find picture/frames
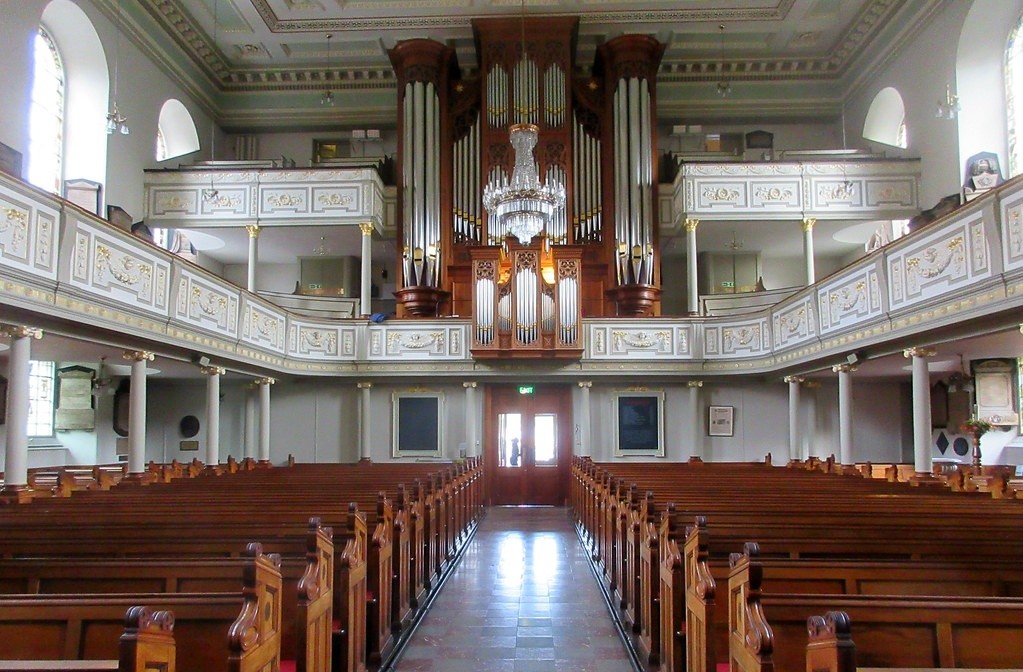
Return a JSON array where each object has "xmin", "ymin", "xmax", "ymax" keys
[
  {"xmin": 708, "ymin": 405, "xmax": 734, "ymax": 438},
  {"xmin": 610, "ymin": 386, "xmax": 666, "ymax": 458},
  {"xmin": 391, "ymin": 390, "xmax": 445, "ymax": 458}
]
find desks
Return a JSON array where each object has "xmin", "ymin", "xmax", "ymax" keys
[
  {"xmin": 349, "ymin": 138, "xmax": 384, "ymax": 158},
  {"xmin": 669, "ymin": 134, "xmax": 705, "ymax": 151}
]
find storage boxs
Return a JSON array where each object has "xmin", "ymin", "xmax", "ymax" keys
[
  {"xmin": 366, "ymin": 129, "xmax": 380, "ymax": 138},
  {"xmin": 689, "ymin": 125, "xmax": 703, "ymax": 134},
  {"xmin": 672, "ymin": 124, "xmax": 687, "ymax": 134},
  {"xmin": 705, "ymin": 134, "xmax": 720, "ymax": 151},
  {"xmin": 352, "ymin": 129, "xmax": 366, "ymax": 138}
]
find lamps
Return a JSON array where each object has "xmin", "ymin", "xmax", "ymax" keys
[
  {"xmin": 381, "ymin": 268, "xmax": 388, "ymax": 279},
  {"xmin": 723, "ymin": 231, "xmax": 743, "ymax": 251},
  {"xmin": 312, "ymin": 236, "xmax": 330, "ymax": 256},
  {"xmin": 717, "ymin": 23, "xmax": 732, "ymax": 98},
  {"xmin": 320, "ymin": 34, "xmax": 335, "ymax": 107},
  {"xmin": 104, "ymin": 0, "xmax": 129, "ymax": 137},
  {"xmin": 935, "ymin": 83, "xmax": 963, "ymax": 120},
  {"xmin": 846, "ymin": 350, "xmax": 867, "ymax": 366},
  {"xmin": 200, "ymin": 0, "xmax": 220, "ymax": 205},
  {"xmin": 480, "ymin": 0, "xmax": 567, "ymax": 249},
  {"xmin": 89, "ymin": 355, "xmax": 116, "ymax": 398}
]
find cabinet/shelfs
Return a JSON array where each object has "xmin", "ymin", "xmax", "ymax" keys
[
  {"xmin": 697, "ymin": 250, "xmax": 762, "ymax": 302},
  {"xmin": 296, "ymin": 255, "xmax": 361, "ymax": 298}
]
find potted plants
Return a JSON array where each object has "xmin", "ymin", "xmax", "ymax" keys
[{"xmin": 959, "ymin": 418, "xmax": 991, "ymax": 465}]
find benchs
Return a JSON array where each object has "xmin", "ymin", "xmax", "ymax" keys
[
  {"xmin": 569, "ymin": 454, "xmax": 1023, "ymax": 672},
  {"xmin": 0, "ymin": 449, "xmax": 484, "ymax": 672}
]
